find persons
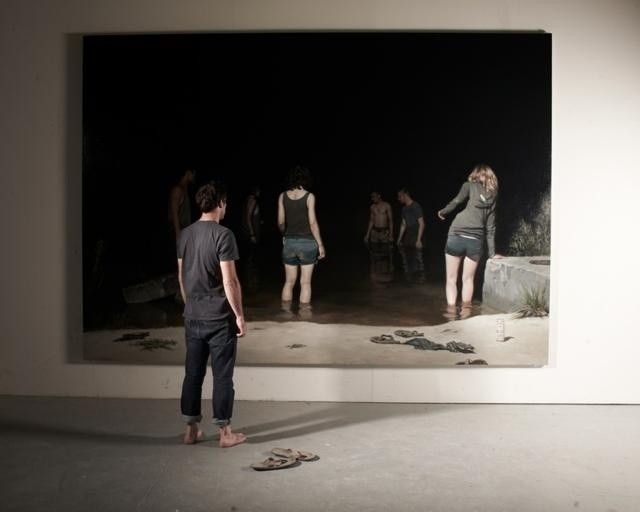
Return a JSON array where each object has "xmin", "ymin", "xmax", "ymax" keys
[
  {"xmin": 364, "ymin": 190, "xmax": 395, "ymax": 243},
  {"xmin": 241, "ymin": 184, "xmax": 264, "ymax": 246},
  {"xmin": 396, "ymin": 188, "xmax": 425, "ymax": 248},
  {"xmin": 167, "ymin": 165, "xmax": 195, "ymax": 238},
  {"xmin": 174, "ymin": 177, "xmax": 247, "ymax": 448},
  {"xmin": 438, "ymin": 162, "xmax": 504, "ymax": 323},
  {"xmin": 277, "ymin": 164, "xmax": 326, "ymax": 303}
]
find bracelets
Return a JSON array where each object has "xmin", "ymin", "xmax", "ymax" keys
[{"xmin": 250, "ymin": 233, "xmax": 256, "ymax": 237}]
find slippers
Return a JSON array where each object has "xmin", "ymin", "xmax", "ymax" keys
[
  {"xmin": 251, "ymin": 455, "xmax": 297, "ymax": 471},
  {"xmin": 395, "ymin": 330, "xmax": 424, "ymax": 337},
  {"xmin": 371, "ymin": 334, "xmax": 400, "ymax": 344},
  {"xmin": 271, "ymin": 447, "xmax": 316, "ymax": 461}
]
